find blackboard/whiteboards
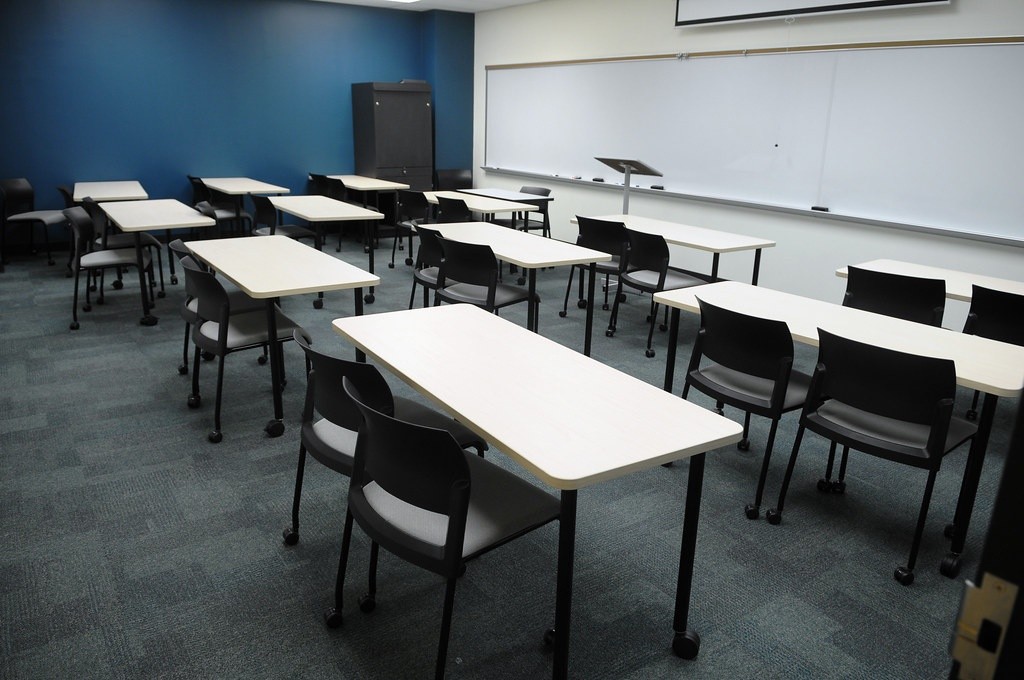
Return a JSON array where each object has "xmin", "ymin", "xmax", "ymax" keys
[{"xmin": 480, "ymin": 37, "xmax": 1024, "ymax": 249}]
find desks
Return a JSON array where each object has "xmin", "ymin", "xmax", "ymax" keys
[
  {"xmin": 182, "ymin": 234, "xmax": 381, "ymax": 439},
  {"xmin": 330, "ymin": 303, "xmax": 745, "ymax": 679},
  {"xmin": 201, "ymin": 177, "xmax": 290, "ymax": 238},
  {"xmin": 73, "ymin": 180, "xmax": 149, "ymax": 201},
  {"xmin": 653, "ymin": 281, "xmax": 1024, "ymax": 580},
  {"xmin": 309, "ymin": 175, "xmax": 410, "ymax": 253},
  {"xmin": 410, "ymin": 221, "xmax": 613, "ymax": 358},
  {"xmin": 267, "ymin": 196, "xmax": 384, "ymax": 309},
  {"xmin": 98, "ymin": 197, "xmax": 216, "ymax": 327},
  {"xmin": 423, "ymin": 187, "xmax": 554, "ymax": 286},
  {"xmin": 571, "ymin": 214, "xmax": 776, "ymax": 328},
  {"xmin": 836, "ymin": 258, "xmax": 1024, "ymax": 304}
]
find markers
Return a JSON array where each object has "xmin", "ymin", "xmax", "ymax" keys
[
  {"xmin": 636, "ymin": 185, "xmax": 650, "ymax": 188},
  {"xmin": 491, "ymin": 167, "xmax": 499, "ymax": 170},
  {"xmin": 571, "ymin": 176, "xmax": 582, "ymax": 179}
]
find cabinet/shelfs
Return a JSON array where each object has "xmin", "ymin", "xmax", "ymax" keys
[{"xmin": 352, "ymin": 82, "xmax": 434, "ymax": 238}]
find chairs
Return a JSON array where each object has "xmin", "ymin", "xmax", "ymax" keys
[
  {"xmin": 1, "ymin": 167, "xmax": 707, "ymax": 442},
  {"xmin": 324, "ymin": 375, "xmax": 563, "ymax": 679},
  {"xmin": 962, "ymin": 283, "xmax": 1024, "ymax": 421},
  {"xmin": 842, "ymin": 266, "xmax": 947, "ymax": 329},
  {"xmin": 659, "ymin": 294, "xmax": 836, "ymax": 520},
  {"xmin": 766, "ymin": 325, "xmax": 976, "ymax": 589},
  {"xmin": 281, "ymin": 326, "xmax": 490, "ymax": 613}
]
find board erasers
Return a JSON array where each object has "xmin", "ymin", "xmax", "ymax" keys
[
  {"xmin": 812, "ymin": 205, "xmax": 829, "ymax": 212},
  {"xmin": 650, "ymin": 184, "xmax": 664, "ymax": 190},
  {"xmin": 593, "ymin": 177, "xmax": 605, "ymax": 182}
]
